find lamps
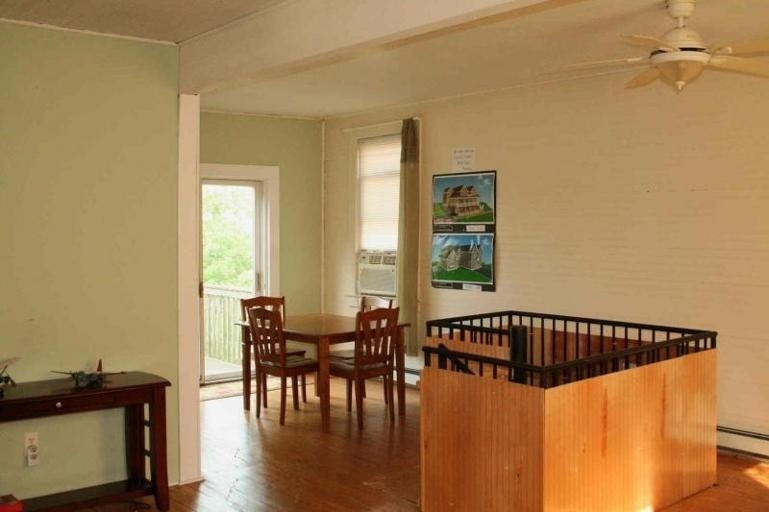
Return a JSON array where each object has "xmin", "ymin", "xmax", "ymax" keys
[{"xmin": 650, "ymin": 50, "xmax": 711, "ymax": 92}]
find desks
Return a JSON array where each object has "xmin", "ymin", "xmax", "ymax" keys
[
  {"xmin": 2, "ymin": 369, "xmax": 170, "ymax": 511},
  {"xmin": 234, "ymin": 314, "xmax": 412, "ymax": 426}
]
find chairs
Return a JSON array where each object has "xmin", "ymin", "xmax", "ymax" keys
[{"xmin": 236, "ymin": 292, "xmax": 401, "ymax": 431}]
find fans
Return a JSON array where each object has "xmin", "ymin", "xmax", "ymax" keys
[{"xmin": 551, "ymin": 0, "xmax": 769, "ymax": 102}]
